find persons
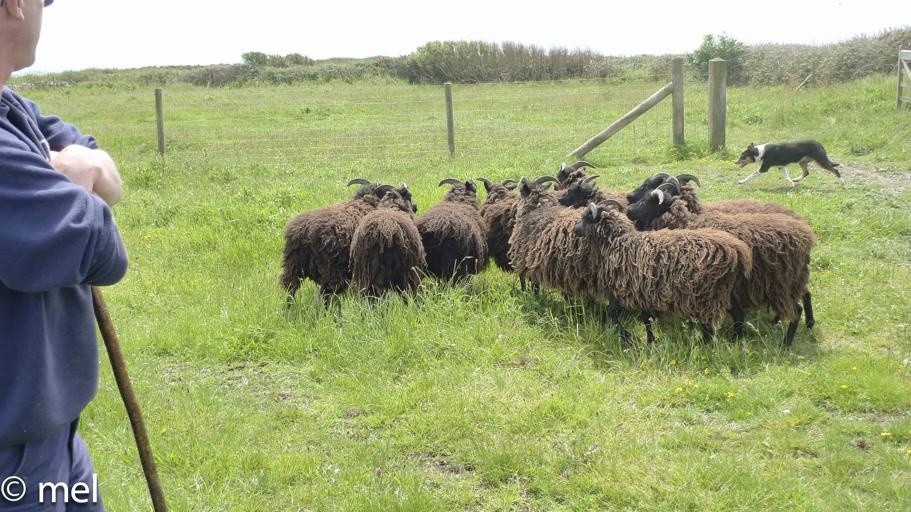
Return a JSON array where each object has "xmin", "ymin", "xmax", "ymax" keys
[{"xmin": 1, "ymin": 0, "xmax": 128, "ymax": 511}]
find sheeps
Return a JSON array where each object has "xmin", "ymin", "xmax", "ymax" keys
[
  {"xmin": 411, "ymin": 177, "xmax": 490, "ymax": 284},
  {"xmin": 349, "ymin": 182, "xmax": 429, "ymax": 309},
  {"xmin": 277, "ymin": 178, "xmax": 385, "ymax": 322},
  {"xmin": 477, "ymin": 161, "xmax": 818, "ymax": 347}
]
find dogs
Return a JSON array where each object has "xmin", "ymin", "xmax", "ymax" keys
[{"xmin": 732, "ymin": 136, "xmax": 844, "ymax": 190}]
